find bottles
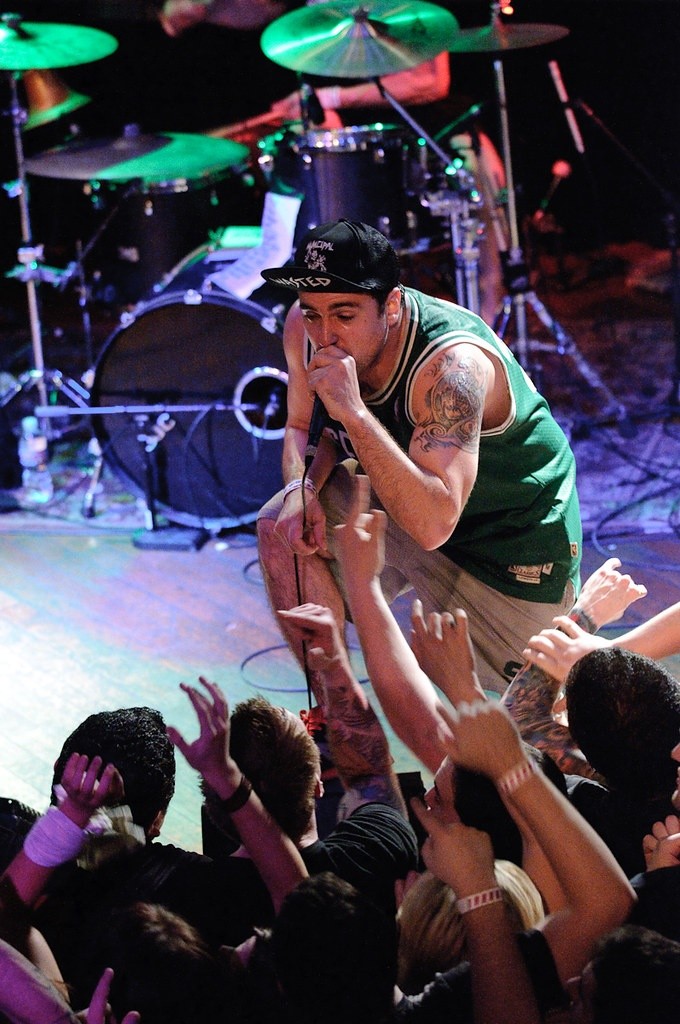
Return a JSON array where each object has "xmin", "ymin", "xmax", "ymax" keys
[{"xmin": 17, "ymin": 415, "xmax": 54, "ymax": 504}]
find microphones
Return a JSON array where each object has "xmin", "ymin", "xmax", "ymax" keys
[
  {"xmin": 548, "ymin": 60, "xmax": 585, "ymax": 155},
  {"xmin": 297, "ymin": 72, "xmax": 325, "ymax": 125},
  {"xmin": 305, "ymin": 393, "xmax": 328, "ymax": 468},
  {"xmin": 532, "ymin": 160, "xmax": 570, "ymax": 221}
]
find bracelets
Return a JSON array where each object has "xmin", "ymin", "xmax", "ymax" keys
[
  {"xmin": 282, "ymin": 479, "xmax": 319, "ymax": 503},
  {"xmin": 220, "ymin": 774, "xmax": 252, "ymax": 811},
  {"xmin": 457, "ymin": 886, "xmax": 501, "ymax": 916},
  {"xmin": 497, "ymin": 755, "xmax": 537, "ymax": 797},
  {"xmin": 332, "ymin": 86, "xmax": 341, "ymax": 108}
]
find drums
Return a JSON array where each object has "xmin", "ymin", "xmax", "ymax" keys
[
  {"xmin": 90, "ymin": 159, "xmax": 254, "ymax": 305},
  {"xmin": 263, "ymin": 125, "xmax": 465, "ymax": 258},
  {"xmin": 88, "ymin": 251, "xmax": 329, "ymax": 530}
]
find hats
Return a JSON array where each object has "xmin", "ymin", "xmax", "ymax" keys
[{"xmin": 258, "ymin": 216, "xmax": 400, "ymax": 297}]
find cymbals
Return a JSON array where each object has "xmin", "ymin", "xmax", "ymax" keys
[
  {"xmin": 0, "ymin": 21, "xmax": 119, "ymax": 71},
  {"xmin": 444, "ymin": 23, "xmax": 569, "ymax": 52},
  {"xmin": 22, "ymin": 127, "xmax": 251, "ymax": 178},
  {"xmin": 260, "ymin": 0, "xmax": 460, "ymax": 79}
]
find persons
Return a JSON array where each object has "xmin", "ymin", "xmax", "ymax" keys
[
  {"xmin": 160, "ymin": 0, "xmax": 511, "ymax": 330},
  {"xmin": 0, "ymin": 556, "xmax": 680, "ymax": 1024},
  {"xmin": 258, "ymin": 222, "xmax": 584, "ymax": 783}
]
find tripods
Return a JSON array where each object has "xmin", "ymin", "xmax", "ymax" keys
[
  {"xmin": 492, "ymin": 54, "xmax": 640, "ymax": 440},
  {"xmin": 0, "ymin": 73, "xmax": 95, "ymax": 456}
]
